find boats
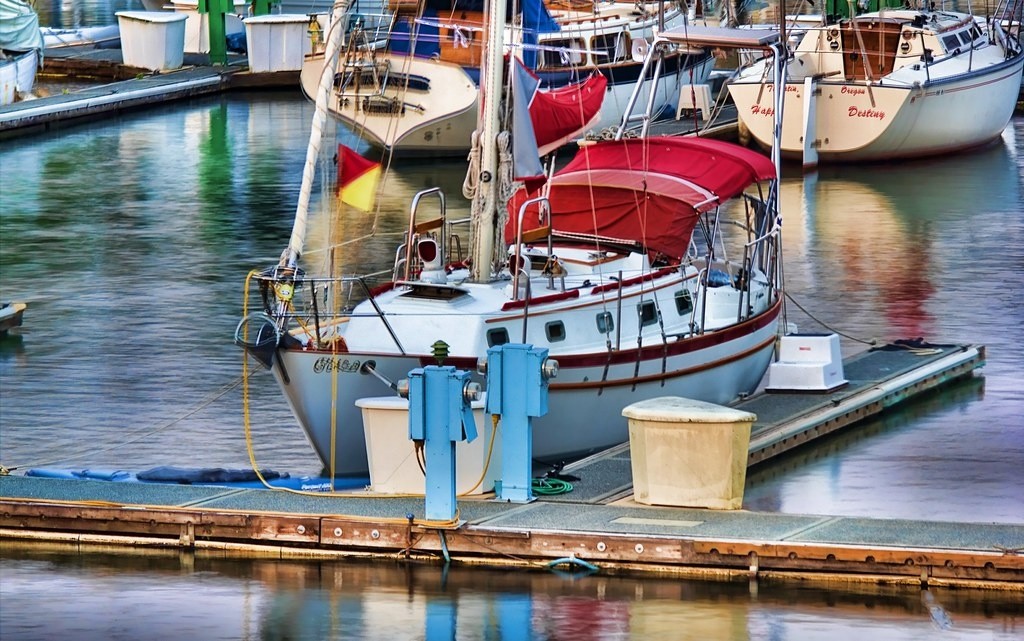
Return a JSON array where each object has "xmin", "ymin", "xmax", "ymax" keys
[
  {"xmin": 291, "ymin": 0, "xmax": 1024, "ymax": 165},
  {"xmin": 0, "ymin": 0, "xmax": 46, "ymax": 108},
  {"xmin": 234, "ymin": 0, "xmax": 789, "ymax": 485},
  {"xmin": 37, "ymin": 23, "xmax": 122, "ymax": 59}
]
